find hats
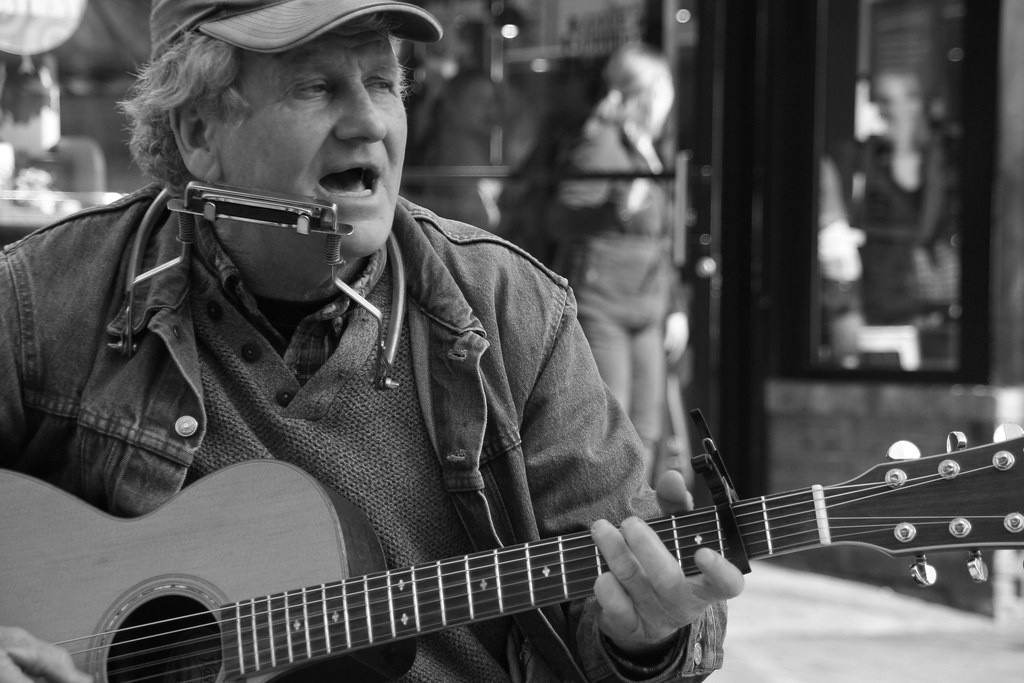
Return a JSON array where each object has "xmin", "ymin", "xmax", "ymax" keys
[{"xmin": 150, "ymin": 0, "xmax": 443, "ymax": 64}]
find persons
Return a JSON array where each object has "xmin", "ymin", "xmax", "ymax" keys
[
  {"xmin": 820, "ymin": 64, "xmax": 949, "ymax": 370},
  {"xmin": 0, "ymin": 0, "xmax": 744, "ymax": 683}
]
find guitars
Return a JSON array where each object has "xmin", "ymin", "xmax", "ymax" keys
[{"xmin": 0, "ymin": 421, "xmax": 1024, "ymax": 683}]
